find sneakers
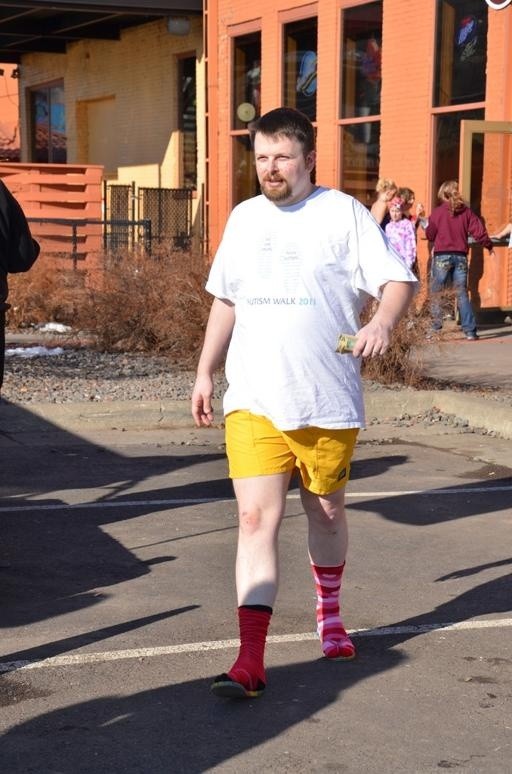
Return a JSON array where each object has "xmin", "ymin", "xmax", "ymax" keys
[{"xmin": 465, "ymin": 329, "xmax": 482, "ymax": 342}]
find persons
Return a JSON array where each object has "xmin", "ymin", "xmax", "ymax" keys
[
  {"xmin": 369, "ymin": 176, "xmax": 399, "ymax": 231},
  {"xmin": 488, "ymin": 221, "xmax": 511, "ymax": 240},
  {"xmin": 0, "ymin": 175, "xmax": 43, "ymax": 388},
  {"xmin": 424, "ymin": 179, "xmax": 498, "ymax": 341},
  {"xmin": 395, "ymin": 186, "xmax": 427, "ymax": 230},
  {"xmin": 190, "ymin": 104, "xmax": 420, "ymax": 701},
  {"xmin": 381, "ymin": 196, "xmax": 419, "ymax": 269}
]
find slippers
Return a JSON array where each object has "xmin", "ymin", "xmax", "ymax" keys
[
  {"xmin": 210, "ymin": 669, "xmax": 268, "ymax": 700},
  {"xmin": 318, "ymin": 635, "xmax": 358, "ymax": 664}
]
